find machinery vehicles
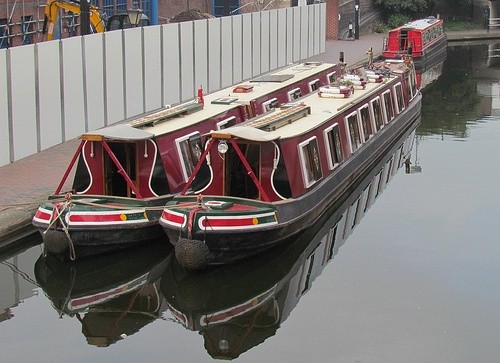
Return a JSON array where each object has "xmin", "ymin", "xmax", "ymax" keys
[{"xmin": 41, "ymin": 0, "xmax": 150, "ymax": 43}]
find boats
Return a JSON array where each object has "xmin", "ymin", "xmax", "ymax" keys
[
  {"xmin": 33, "ymin": 237, "xmax": 176, "ymax": 349},
  {"xmin": 31, "ymin": 60, "xmax": 348, "ymax": 262},
  {"xmin": 411, "ymin": 34, "xmax": 449, "ymax": 90},
  {"xmin": 159, "ymin": 115, "xmax": 424, "ymax": 361},
  {"xmin": 381, "ymin": 14, "xmax": 447, "ymax": 61},
  {"xmin": 159, "ymin": 58, "xmax": 423, "ymax": 275}
]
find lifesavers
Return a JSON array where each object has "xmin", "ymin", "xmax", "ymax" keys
[
  {"xmin": 353, "ymin": 86, "xmax": 366, "ymax": 90},
  {"xmin": 318, "ymin": 86, "xmax": 354, "ymax": 93},
  {"xmin": 367, "ymin": 79, "xmax": 383, "ymax": 83},
  {"xmin": 318, "ymin": 92, "xmax": 351, "ymax": 98},
  {"xmin": 338, "ymin": 75, "xmax": 367, "ymax": 85},
  {"xmin": 365, "ymin": 70, "xmax": 383, "ymax": 79}
]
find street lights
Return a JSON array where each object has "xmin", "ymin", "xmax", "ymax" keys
[{"xmin": 127, "ymin": 1, "xmax": 144, "ymax": 28}]
